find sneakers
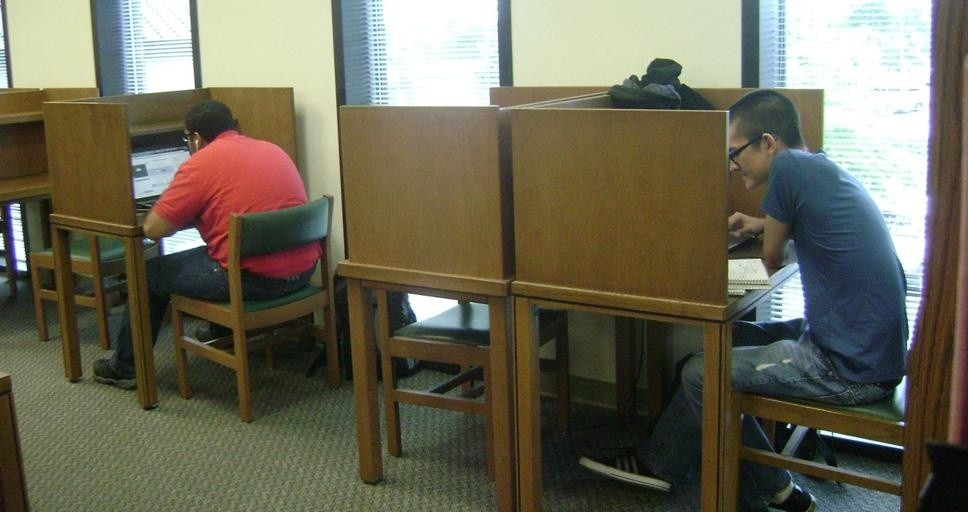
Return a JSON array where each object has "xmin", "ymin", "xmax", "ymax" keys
[
  {"xmin": 579, "ymin": 453, "xmax": 672, "ymax": 494},
  {"xmin": 92, "ymin": 358, "xmax": 136, "ymax": 389},
  {"xmin": 195, "ymin": 326, "xmax": 213, "ymax": 342},
  {"xmin": 760, "ymin": 481, "xmax": 816, "ymax": 512}
]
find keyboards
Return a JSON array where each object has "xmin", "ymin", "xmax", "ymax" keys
[{"xmin": 728, "ymin": 236, "xmax": 750, "ymax": 254}]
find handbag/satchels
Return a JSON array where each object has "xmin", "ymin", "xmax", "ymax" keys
[{"xmin": 333, "ymin": 271, "xmax": 421, "ymax": 380}]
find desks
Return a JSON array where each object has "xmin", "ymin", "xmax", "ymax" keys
[
  {"xmin": 0, "ymin": 84, "xmax": 298, "ymax": 391},
  {"xmin": 331, "ymin": 84, "xmax": 826, "ymax": 510}
]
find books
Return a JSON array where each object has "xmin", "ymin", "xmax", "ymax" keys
[{"xmin": 727, "ymin": 257, "xmax": 772, "ymax": 297}]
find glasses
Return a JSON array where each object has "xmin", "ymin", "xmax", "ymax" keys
[
  {"xmin": 727, "ymin": 134, "xmax": 763, "ymax": 162},
  {"xmin": 182, "ymin": 133, "xmax": 193, "ymax": 142}
]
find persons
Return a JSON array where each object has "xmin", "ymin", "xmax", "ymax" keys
[
  {"xmin": 578, "ymin": 87, "xmax": 909, "ymax": 512},
  {"xmin": 92, "ymin": 99, "xmax": 323, "ymax": 390}
]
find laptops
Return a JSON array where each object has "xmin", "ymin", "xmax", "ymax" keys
[{"xmin": 131, "ymin": 144, "xmax": 191, "ymax": 209}]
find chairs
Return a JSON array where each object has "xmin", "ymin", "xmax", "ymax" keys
[
  {"xmin": 167, "ymin": 191, "xmax": 344, "ymax": 424},
  {"xmin": 732, "ymin": 224, "xmax": 932, "ymax": 512}
]
